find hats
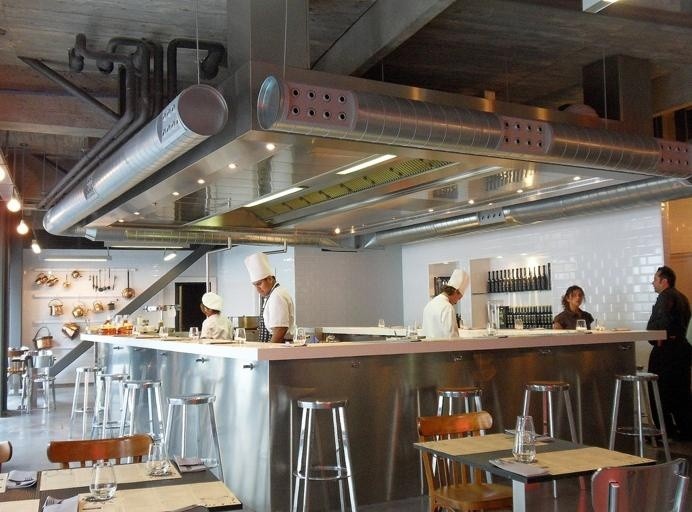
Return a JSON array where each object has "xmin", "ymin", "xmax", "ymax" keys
[
  {"xmin": 447, "ymin": 269, "xmax": 469, "ymax": 295},
  {"xmin": 202, "ymin": 292, "xmax": 222, "ymax": 310},
  {"xmin": 244, "ymin": 252, "xmax": 274, "ymax": 282}
]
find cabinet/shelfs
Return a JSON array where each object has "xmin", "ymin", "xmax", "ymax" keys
[{"xmin": 471, "ymin": 251, "xmax": 553, "ymax": 329}]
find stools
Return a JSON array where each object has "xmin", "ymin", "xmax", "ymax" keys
[
  {"xmin": 165, "ymin": 394, "xmax": 224, "ymax": 481},
  {"xmin": 91, "ymin": 374, "xmax": 130, "ymax": 439},
  {"xmin": 523, "ymin": 381, "xmax": 586, "ymax": 498},
  {"xmin": 70, "ymin": 365, "xmax": 101, "ymax": 433},
  {"xmin": 292, "ymin": 398, "xmax": 359, "ymax": 512},
  {"xmin": 119, "ymin": 380, "xmax": 165, "ymax": 464},
  {"xmin": 431, "ymin": 386, "xmax": 494, "ymax": 483},
  {"xmin": 609, "ymin": 373, "xmax": 671, "ymax": 462}
]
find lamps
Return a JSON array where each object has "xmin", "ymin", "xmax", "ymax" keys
[
  {"xmin": 0, "ymin": 131, "xmax": 41, "ymax": 253},
  {"xmin": 42, "ymin": 246, "xmax": 111, "ymax": 261},
  {"xmin": 162, "ymin": 248, "xmax": 176, "ymax": 261}
]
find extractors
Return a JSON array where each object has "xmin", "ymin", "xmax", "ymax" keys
[{"xmin": 250, "ymin": 155, "xmax": 455, "ymax": 223}]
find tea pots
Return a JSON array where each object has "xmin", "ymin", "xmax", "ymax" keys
[{"xmin": 72, "ymin": 300, "xmax": 104, "ymax": 318}]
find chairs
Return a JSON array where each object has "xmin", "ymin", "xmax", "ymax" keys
[
  {"xmin": 47, "ymin": 434, "xmax": 154, "ymax": 469},
  {"xmin": 591, "ymin": 458, "xmax": 690, "ymax": 512},
  {"xmin": 416, "ymin": 411, "xmax": 513, "ymax": 512},
  {"xmin": 21, "ymin": 349, "xmax": 56, "ymax": 411},
  {"xmin": 0, "ymin": 441, "xmax": 12, "ymax": 472},
  {"xmin": 7, "ymin": 350, "xmax": 27, "ymax": 380}
]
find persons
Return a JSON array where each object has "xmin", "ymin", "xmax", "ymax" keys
[
  {"xmin": 198, "ymin": 291, "xmax": 236, "ymax": 341},
  {"xmin": 421, "ymin": 269, "xmax": 469, "ymax": 339},
  {"xmin": 551, "ymin": 284, "xmax": 596, "ymax": 329},
  {"xmin": 645, "ymin": 266, "xmax": 691, "ymax": 447},
  {"xmin": 244, "ymin": 252, "xmax": 297, "ymax": 343}
]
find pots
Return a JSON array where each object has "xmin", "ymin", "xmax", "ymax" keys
[
  {"xmin": 34, "ymin": 336, "xmax": 53, "ymax": 348},
  {"xmin": 35, "ymin": 270, "xmax": 83, "ymax": 288},
  {"xmin": 49, "ymin": 305, "xmax": 64, "ymax": 316},
  {"xmin": 122, "ymin": 271, "xmax": 135, "ymax": 300}
]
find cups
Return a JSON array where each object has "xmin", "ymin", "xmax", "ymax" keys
[
  {"xmin": 314, "ymin": 327, "xmax": 323, "ymax": 341},
  {"xmin": 378, "ymin": 319, "xmax": 385, "ymax": 328},
  {"xmin": 595, "ymin": 317, "xmax": 605, "ymax": 330},
  {"xmin": 189, "ymin": 327, "xmax": 199, "ymax": 339},
  {"xmin": 575, "ymin": 319, "xmax": 587, "ymax": 331},
  {"xmin": 159, "ymin": 327, "xmax": 169, "ymax": 339},
  {"xmin": 234, "ymin": 328, "xmax": 247, "ymax": 341},
  {"xmin": 486, "ymin": 322, "xmax": 497, "ymax": 336},
  {"xmin": 406, "ymin": 321, "xmax": 418, "ymax": 339},
  {"xmin": 293, "ymin": 327, "xmax": 306, "ymax": 347}
]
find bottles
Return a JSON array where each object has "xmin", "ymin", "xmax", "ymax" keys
[
  {"xmin": 116, "ymin": 314, "xmax": 129, "ymax": 325},
  {"xmin": 145, "ymin": 442, "xmax": 170, "ymax": 475},
  {"xmin": 499, "ymin": 307, "xmax": 552, "ymax": 328},
  {"xmin": 456, "ymin": 313, "xmax": 461, "ymax": 328},
  {"xmin": 89, "ymin": 462, "xmax": 119, "ymax": 500},
  {"xmin": 486, "ymin": 262, "xmax": 550, "ymax": 293},
  {"xmin": 512, "ymin": 416, "xmax": 536, "ymax": 463},
  {"xmin": 435, "ymin": 277, "xmax": 450, "ymax": 296}
]
